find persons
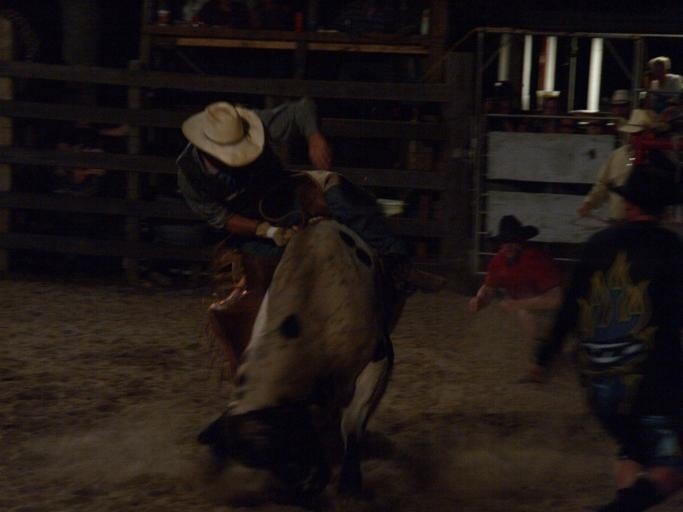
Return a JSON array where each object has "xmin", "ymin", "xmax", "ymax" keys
[
  {"xmin": 50, "ymin": 0, "xmax": 682, "ymax": 384},
  {"xmin": 172, "ymin": 89, "xmax": 447, "ymax": 313},
  {"xmin": 513, "ymin": 174, "xmax": 683, "ymax": 511}
]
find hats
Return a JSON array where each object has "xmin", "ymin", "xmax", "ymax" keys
[
  {"xmin": 181, "ymin": 103, "xmax": 266, "ymax": 166},
  {"xmin": 609, "ymin": 89, "xmax": 630, "ymax": 104},
  {"xmin": 606, "ymin": 163, "xmax": 681, "ymax": 206},
  {"xmin": 617, "ymin": 108, "xmax": 670, "ymax": 134},
  {"xmin": 486, "ymin": 215, "xmax": 539, "ymax": 243}
]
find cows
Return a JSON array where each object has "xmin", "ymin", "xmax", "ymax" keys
[{"xmin": 198, "ymin": 214, "xmax": 394, "ymax": 512}]
